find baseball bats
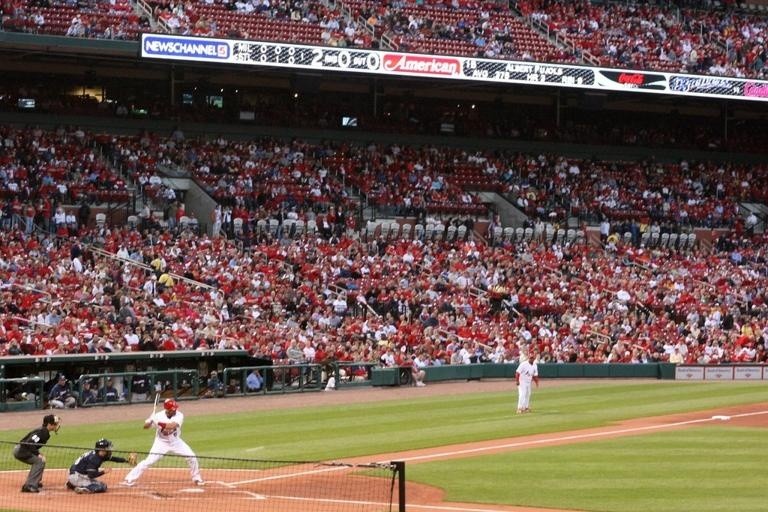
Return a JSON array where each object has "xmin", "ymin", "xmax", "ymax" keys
[{"xmin": 152, "ymin": 392, "xmax": 160, "ymax": 417}]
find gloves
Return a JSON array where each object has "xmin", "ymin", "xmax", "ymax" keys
[{"xmin": 145, "ymin": 413, "xmax": 159, "ymax": 427}]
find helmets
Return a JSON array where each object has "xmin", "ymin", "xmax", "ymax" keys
[
  {"xmin": 43, "ymin": 414, "xmax": 59, "ymax": 428},
  {"xmin": 94, "ymin": 439, "xmax": 114, "ymax": 452}
]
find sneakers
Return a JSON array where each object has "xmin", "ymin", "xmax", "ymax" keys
[
  {"xmin": 74, "ymin": 486, "xmax": 90, "ymax": 494},
  {"xmin": 124, "ymin": 480, "xmax": 137, "ymax": 487},
  {"xmin": 191, "ymin": 480, "xmax": 208, "ymax": 487},
  {"xmin": 22, "ymin": 482, "xmax": 42, "ymax": 493},
  {"xmin": 415, "ymin": 381, "xmax": 426, "ymax": 387},
  {"xmin": 516, "ymin": 408, "xmax": 531, "ymax": 414}
]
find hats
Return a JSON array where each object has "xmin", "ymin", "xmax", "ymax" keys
[
  {"xmin": 105, "ymin": 377, "xmax": 111, "ymax": 382},
  {"xmin": 163, "ymin": 399, "xmax": 179, "ymax": 410},
  {"xmin": 59, "ymin": 375, "xmax": 65, "ymax": 379},
  {"xmin": 529, "ymin": 352, "xmax": 536, "ymax": 359},
  {"xmin": 83, "ymin": 379, "xmax": 90, "ymax": 384}
]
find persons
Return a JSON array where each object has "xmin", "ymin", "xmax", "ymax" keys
[
  {"xmin": 515, "ymin": 354, "xmax": 539, "ymax": 414},
  {"xmin": 119, "ymin": 399, "xmax": 205, "ymax": 487},
  {"xmin": 0, "ymin": 1, "xmax": 768, "ymax": 410},
  {"xmin": 13, "ymin": 415, "xmax": 59, "ymax": 492},
  {"xmin": 67, "ymin": 438, "xmax": 129, "ymax": 494}
]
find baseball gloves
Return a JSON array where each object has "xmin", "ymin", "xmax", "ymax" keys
[{"xmin": 127, "ymin": 452, "xmax": 138, "ymax": 467}]
